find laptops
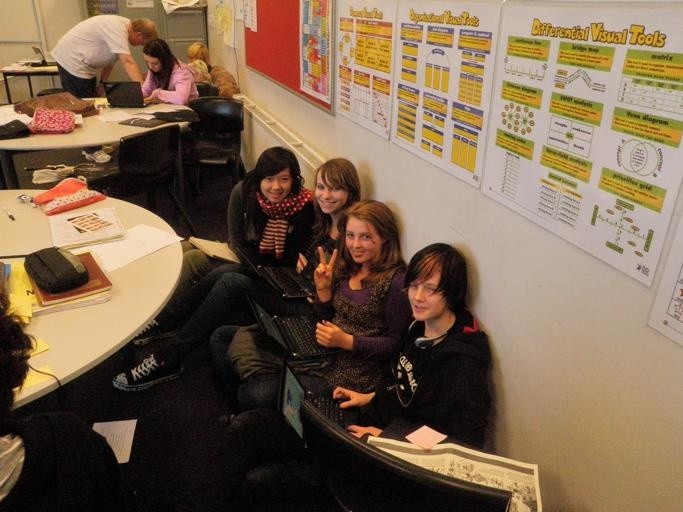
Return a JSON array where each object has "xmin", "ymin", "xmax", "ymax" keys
[
  {"xmin": 243, "ymin": 292, "xmax": 344, "ymax": 361},
  {"xmin": 234, "ymin": 246, "xmax": 317, "ymax": 298},
  {"xmin": 31, "ymin": 46, "xmax": 57, "ymax": 66},
  {"xmin": 102, "ymin": 81, "xmax": 150, "ymax": 108},
  {"xmin": 276, "ymin": 360, "xmax": 346, "ymax": 450}
]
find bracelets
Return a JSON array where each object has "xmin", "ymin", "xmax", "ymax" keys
[{"xmin": 100, "ymin": 79, "xmax": 107, "ymax": 85}]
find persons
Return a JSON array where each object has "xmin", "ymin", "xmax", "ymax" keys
[
  {"xmin": 119, "ymin": 38, "xmax": 199, "ymax": 178},
  {"xmin": 187, "ymin": 42, "xmax": 212, "ymax": 74},
  {"xmin": 197, "ymin": 242, "xmax": 491, "ymax": 510},
  {"xmin": 112, "ymin": 159, "xmax": 361, "ymax": 393},
  {"xmin": 50, "ymin": 16, "xmax": 158, "ymax": 164},
  {"xmin": 210, "ymin": 200, "xmax": 412, "ymax": 398},
  {"xmin": 0, "ymin": 282, "xmax": 123, "ymax": 509},
  {"xmin": 174, "ymin": 145, "xmax": 309, "ymax": 295}
]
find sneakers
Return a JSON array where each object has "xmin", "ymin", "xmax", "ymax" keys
[
  {"xmin": 299, "ymin": 463, "xmax": 320, "ymax": 481},
  {"xmin": 133, "ymin": 320, "xmax": 179, "ymax": 346},
  {"xmin": 246, "ymin": 461, "xmax": 297, "ymax": 486},
  {"xmin": 82, "ymin": 145, "xmax": 113, "ymax": 156},
  {"xmin": 86, "ymin": 150, "xmax": 112, "ymax": 163},
  {"xmin": 112, "ymin": 354, "xmax": 184, "ymax": 391}
]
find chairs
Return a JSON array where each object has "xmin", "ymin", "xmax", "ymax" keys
[
  {"xmin": 118, "ymin": 123, "xmax": 180, "ymax": 212},
  {"xmin": 183, "ymin": 98, "xmax": 244, "ymax": 187},
  {"xmin": 196, "ymin": 84, "xmax": 219, "ymax": 96}
]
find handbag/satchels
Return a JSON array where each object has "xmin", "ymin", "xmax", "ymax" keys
[
  {"xmin": 25, "ymin": 246, "xmax": 88, "ymax": 294},
  {"xmin": 33, "ymin": 176, "xmax": 106, "ymax": 216},
  {"xmin": 26, "ymin": 108, "xmax": 75, "ymax": 133},
  {"xmin": 14, "ymin": 92, "xmax": 99, "ymax": 117}
]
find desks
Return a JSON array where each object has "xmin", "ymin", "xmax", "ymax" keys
[
  {"xmin": 0, "ymin": 189, "xmax": 184, "ymax": 412},
  {"xmin": 0, "ymin": 60, "xmax": 61, "ymax": 104},
  {"xmin": 0, "ymin": 98, "xmax": 195, "ymax": 203}
]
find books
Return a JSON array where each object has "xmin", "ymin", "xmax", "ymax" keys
[
  {"xmin": 119, "ymin": 109, "xmax": 199, "ymax": 128},
  {"xmin": 189, "ymin": 236, "xmax": 243, "ymax": 265},
  {"xmin": 0, "ymin": 204, "xmax": 124, "ymax": 318}
]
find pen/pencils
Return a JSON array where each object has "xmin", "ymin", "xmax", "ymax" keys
[
  {"xmin": 1, "ymin": 204, "xmax": 15, "ymax": 221},
  {"xmin": 24, "ymin": 166, "xmax": 57, "ymax": 171}
]
